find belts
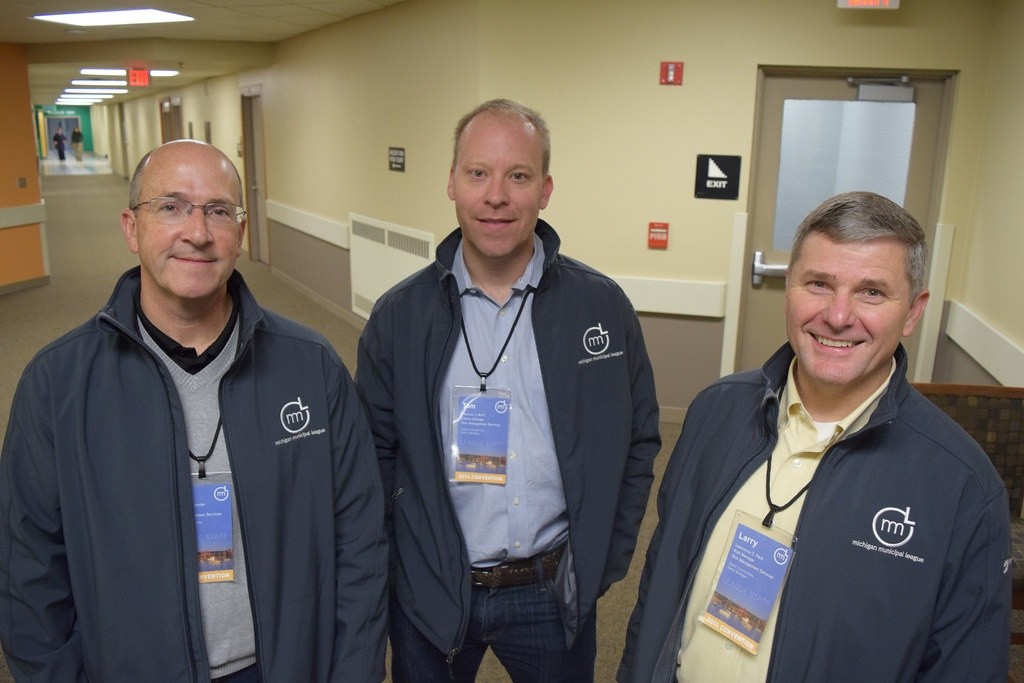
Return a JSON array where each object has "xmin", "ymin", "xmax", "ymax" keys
[{"xmin": 471, "ymin": 555, "xmax": 561, "ymax": 587}]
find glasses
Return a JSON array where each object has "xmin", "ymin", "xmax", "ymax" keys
[{"xmin": 132, "ymin": 196, "xmax": 248, "ymax": 226}]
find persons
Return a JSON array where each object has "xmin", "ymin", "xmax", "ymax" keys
[
  {"xmin": 53, "ymin": 128, "xmax": 67, "ymax": 161},
  {"xmin": 0, "ymin": 136, "xmax": 392, "ymax": 683},
  {"xmin": 610, "ymin": 189, "xmax": 1015, "ymax": 683},
  {"xmin": 71, "ymin": 126, "xmax": 83, "ymax": 161},
  {"xmin": 349, "ymin": 96, "xmax": 664, "ymax": 683}
]
